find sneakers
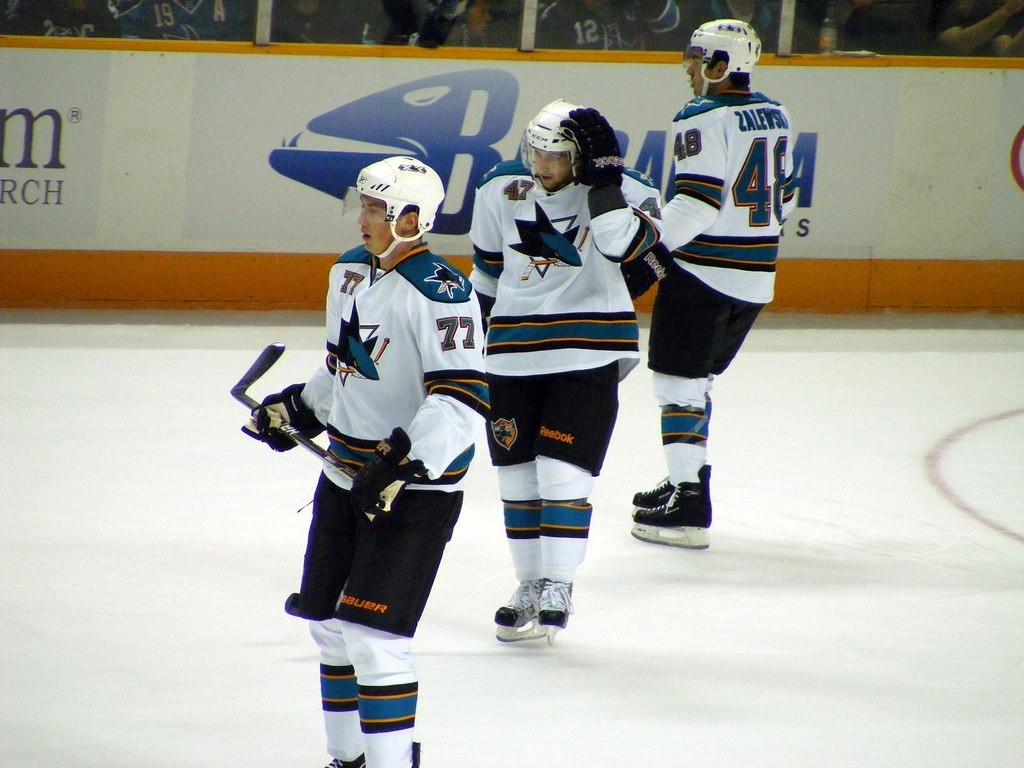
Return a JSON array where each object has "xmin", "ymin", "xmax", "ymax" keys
[
  {"xmin": 495, "ymin": 579, "xmax": 546, "ymax": 641},
  {"xmin": 632, "ymin": 464, "xmax": 713, "ymax": 550},
  {"xmin": 541, "ymin": 578, "xmax": 573, "ymax": 646},
  {"xmin": 632, "ymin": 476, "xmax": 678, "ymax": 511}
]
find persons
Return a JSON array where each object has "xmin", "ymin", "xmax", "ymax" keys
[
  {"xmin": 270, "ymin": 0, "xmax": 525, "ymax": 48},
  {"xmin": 534, "ymin": 0, "xmax": 782, "ymax": 54},
  {"xmin": 242, "ymin": 156, "xmax": 491, "ymax": 768},
  {"xmin": 792, "ymin": 0, "xmax": 1024, "ymax": 58},
  {"xmin": 468, "ymin": 99, "xmax": 665, "ymax": 646},
  {"xmin": 620, "ymin": 20, "xmax": 795, "ymax": 550},
  {"xmin": 0, "ymin": 0, "xmax": 258, "ymax": 44}
]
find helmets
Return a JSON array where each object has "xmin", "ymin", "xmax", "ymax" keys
[
  {"xmin": 681, "ymin": 18, "xmax": 762, "ymax": 84},
  {"xmin": 521, "ymin": 97, "xmax": 582, "ymax": 177},
  {"xmin": 342, "ymin": 156, "xmax": 445, "ymax": 243}
]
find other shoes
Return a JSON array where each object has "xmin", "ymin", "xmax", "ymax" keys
[{"xmin": 326, "ymin": 740, "xmax": 420, "ymax": 768}]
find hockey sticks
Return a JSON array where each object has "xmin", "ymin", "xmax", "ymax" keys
[{"xmin": 228, "ymin": 341, "xmax": 402, "ymax": 515}]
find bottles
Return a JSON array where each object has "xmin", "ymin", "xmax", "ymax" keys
[{"xmin": 818, "ymin": 0, "xmax": 837, "ymax": 53}]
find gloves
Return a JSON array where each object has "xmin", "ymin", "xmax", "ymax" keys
[
  {"xmin": 350, "ymin": 428, "xmax": 428, "ymax": 528},
  {"xmin": 620, "ymin": 243, "xmax": 672, "ymax": 299},
  {"xmin": 561, "ymin": 108, "xmax": 622, "ymax": 186},
  {"xmin": 241, "ymin": 383, "xmax": 329, "ymax": 452}
]
